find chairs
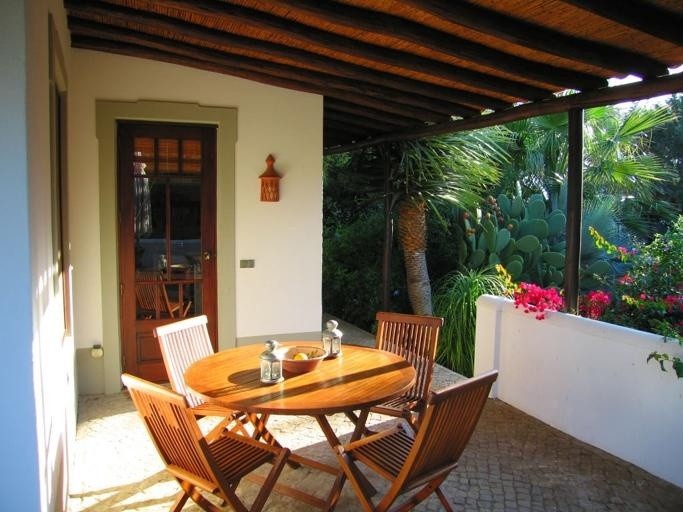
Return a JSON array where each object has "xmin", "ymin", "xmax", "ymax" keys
[
  {"xmin": 153, "ymin": 315, "xmax": 250, "ymax": 443},
  {"xmin": 345, "ymin": 311, "xmax": 444, "ymax": 437},
  {"xmin": 334, "ymin": 369, "xmax": 499, "ymax": 512},
  {"xmin": 135, "ymin": 271, "xmax": 194, "ymax": 320},
  {"xmin": 121, "ymin": 373, "xmax": 290, "ymax": 512}
]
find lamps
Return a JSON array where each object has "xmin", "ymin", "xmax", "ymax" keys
[{"xmin": 259, "ymin": 154, "xmax": 282, "ymax": 202}]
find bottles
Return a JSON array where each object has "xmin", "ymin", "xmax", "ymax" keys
[
  {"xmin": 257, "ymin": 339, "xmax": 286, "ymax": 383},
  {"xmin": 321, "ymin": 320, "xmax": 345, "ymax": 357}
]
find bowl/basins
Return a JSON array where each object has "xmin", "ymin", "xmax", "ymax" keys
[{"xmin": 273, "ymin": 345, "xmax": 328, "ymax": 372}]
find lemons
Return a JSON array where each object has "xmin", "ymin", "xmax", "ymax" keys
[{"xmin": 293, "ymin": 353, "xmax": 308, "ymax": 361}]
